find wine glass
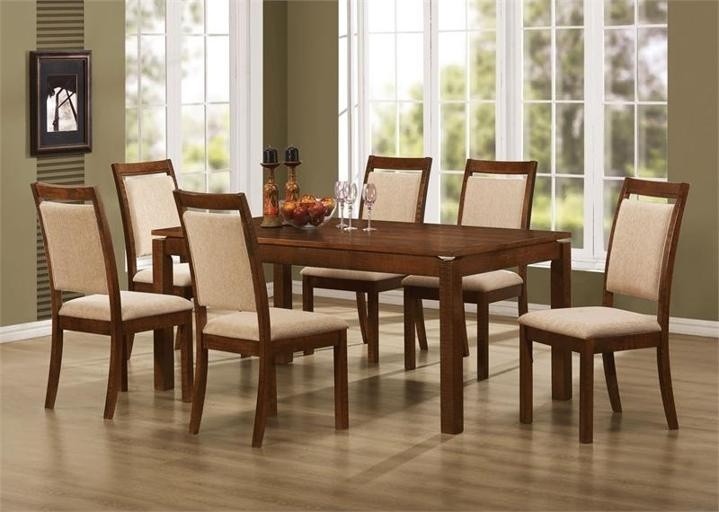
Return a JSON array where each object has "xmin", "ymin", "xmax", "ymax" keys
[{"xmin": 335, "ymin": 181, "xmax": 377, "ymax": 231}]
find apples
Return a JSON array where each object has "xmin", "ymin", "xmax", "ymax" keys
[{"xmin": 282, "ymin": 193, "xmax": 335, "ymax": 227}]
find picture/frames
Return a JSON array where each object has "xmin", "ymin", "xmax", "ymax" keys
[{"xmin": 28, "ymin": 50, "xmax": 92, "ymax": 158}]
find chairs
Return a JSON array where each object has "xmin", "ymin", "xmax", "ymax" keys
[
  {"xmin": 300, "ymin": 155, "xmax": 432, "ymax": 363},
  {"xmin": 172, "ymin": 190, "xmax": 350, "ymax": 446},
  {"xmin": 108, "ymin": 161, "xmax": 192, "ymax": 359},
  {"xmin": 29, "ymin": 181, "xmax": 194, "ymax": 418},
  {"xmin": 401, "ymin": 159, "xmax": 538, "ymax": 369},
  {"xmin": 518, "ymin": 177, "xmax": 690, "ymax": 444}
]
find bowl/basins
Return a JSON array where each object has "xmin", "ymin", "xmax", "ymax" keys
[{"xmin": 279, "ymin": 200, "xmax": 338, "ymax": 230}]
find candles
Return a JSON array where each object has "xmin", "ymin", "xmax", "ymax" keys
[
  {"xmin": 285, "ymin": 145, "xmax": 299, "ymax": 161},
  {"xmin": 263, "ymin": 145, "xmax": 278, "ymax": 163}
]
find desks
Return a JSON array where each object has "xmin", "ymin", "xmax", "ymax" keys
[{"xmin": 150, "ymin": 217, "xmax": 571, "ymax": 435}]
